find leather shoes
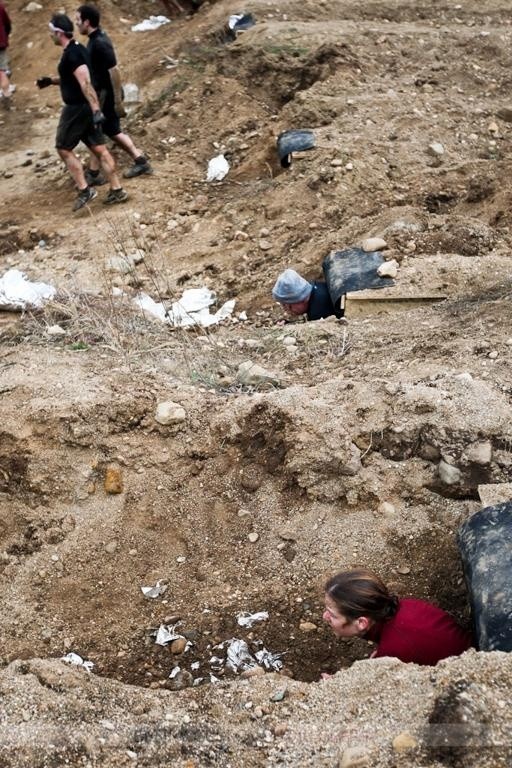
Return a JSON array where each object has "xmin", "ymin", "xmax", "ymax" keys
[
  {"xmin": 123, "ymin": 160, "xmax": 153, "ymax": 178},
  {"xmin": 74, "ymin": 172, "xmax": 105, "ymax": 189}
]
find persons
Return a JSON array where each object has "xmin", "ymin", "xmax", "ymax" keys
[
  {"xmin": 72, "ymin": 4, "xmax": 154, "ymax": 192},
  {"xmin": 270, "ymin": 267, "xmax": 345, "ymax": 327},
  {"xmin": 34, "ymin": 13, "xmax": 129, "ymax": 212},
  {"xmin": 0, "ymin": 0, "xmax": 14, "ymax": 98},
  {"xmin": 319, "ymin": 566, "xmax": 479, "ymax": 681}
]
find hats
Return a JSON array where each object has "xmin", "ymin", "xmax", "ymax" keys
[{"xmin": 272, "ymin": 269, "xmax": 313, "ymax": 303}]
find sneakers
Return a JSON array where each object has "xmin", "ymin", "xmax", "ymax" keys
[
  {"xmin": 0, "ymin": 93, "xmax": 16, "ymax": 104},
  {"xmin": 1, "ymin": 84, "xmax": 17, "ymax": 97},
  {"xmin": 103, "ymin": 189, "xmax": 128, "ymax": 204},
  {"xmin": 72, "ymin": 187, "xmax": 98, "ymax": 211}
]
